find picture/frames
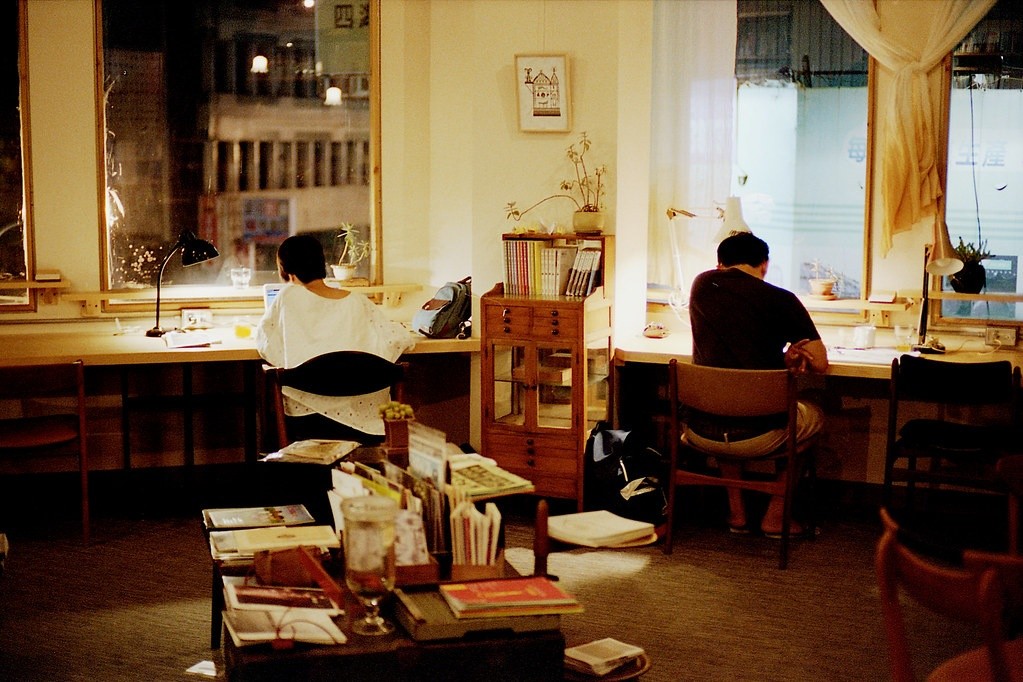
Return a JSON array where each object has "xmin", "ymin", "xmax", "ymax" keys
[{"xmin": 514, "ymin": 52, "xmax": 571, "ymax": 133}]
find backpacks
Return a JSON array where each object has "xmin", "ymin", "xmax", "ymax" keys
[
  {"xmin": 582, "ymin": 419, "xmax": 671, "ymax": 539},
  {"xmin": 413, "ymin": 276, "xmax": 472, "ymax": 339}
]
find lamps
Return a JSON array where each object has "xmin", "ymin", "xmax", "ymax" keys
[
  {"xmin": 912, "ymin": 219, "xmax": 966, "ymax": 353},
  {"xmin": 146, "ymin": 231, "xmax": 219, "ymax": 336},
  {"xmin": 659, "ymin": 195, "xmax": 753, "ymax": 323}
]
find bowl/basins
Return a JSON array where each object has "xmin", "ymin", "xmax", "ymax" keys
[{"xmin": 809, "ymin": 279, "xmax": 834, "ymax": 295}]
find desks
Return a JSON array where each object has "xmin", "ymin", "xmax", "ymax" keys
[
  {"xmin": 0, "ymin": 311, "xmax": 474, "ymax": 518},
  {"xmin": 611, "ymin": 326, "xmax": 1023, "ymax": 389}
]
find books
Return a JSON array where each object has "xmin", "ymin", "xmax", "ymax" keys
[
  {"xmin": 202, "ymin": 503, "xmax": 340, "ymax": 559},
  {"xmin": 451, "ymin": 462, "xmax": 535, "ymax": 502},
  {"xmin": 440, "ymin": 576, "xmax": 585, "ymax": 620},
  {"xmin": 547, "ymin": 510, "xmax": 654, "ymax": 547},
  {"xmin": 327, "ymin": 461, "xmax": 415, "ymax": 540},
  {"xmin": 222, "ymin": 575, "xmax": 348, "ymax": 644},
  {"xmin": 256, "ymin": 440, "xmax": 361, "ymax": 466},
  {"xmin": 503, "ymin": 239, "xmax": 552, "ymax": 295},
  {"xmin": 566, "ymin": 249, "xmax": 601, "ymax": 296},
  {"xmin": 381, "ymin": 459, "xmax": 502, "ymax": 564},
  {"xmin": 564, "ymin": 637, "xmax": 645, "ymax": 676},
  {"xmin": 541, "ymin": 247, "xmax": 577, "ymax": 295}
]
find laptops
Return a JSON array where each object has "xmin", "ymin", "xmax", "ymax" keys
[{"xmin": 264, "ymin": 283, "xmax": 341, "ymax": 310}]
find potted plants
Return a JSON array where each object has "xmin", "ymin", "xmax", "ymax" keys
[
  {"xmin": 502, "ymin": 131, "xmax": 611, "ymax": 235},
  {"xmin": 949, "ymin": 236, "xmax": 997, "ymax": 294},
  {"xmin": 329, "ymin": 221, "xmax": 371, "ymax": 281}
]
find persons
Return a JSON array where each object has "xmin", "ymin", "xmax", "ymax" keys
[
  {"xmin": 682, "ymin": 231, "xmax": 828, "ymax": 539},
  {"xmin": 254, "ymin": 236, "xmax": 415, "ymax": 447}
]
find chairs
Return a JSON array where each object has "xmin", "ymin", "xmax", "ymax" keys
[
  {"xmin": 663, "ymin": 358, "xmax": 822, "ymax": 573},
  {"xmin": 0, "ymin": 359, "xmax": 90, "ymax": 549},
  {"xmin": 874, "ymin": 506, "xmax": 1023, "ymax": 682},
  {"xmin": 884, "ymin": 354, "xmax": 1023, "ymax": 529}
]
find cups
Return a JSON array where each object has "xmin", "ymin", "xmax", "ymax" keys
[
  {"xmin": 231, "ymin": 268, "xmax": 252, "ymax": 289},
  {"xmin": 233, "ymin": 315, "xmax": 251, "ymax": 338},
  {"xmin": 853, "ymin": 326, "xmax": 876, "ymax": 348},
  {"xmin": 894, "ymin": 323, "xmax": 914, "ymax": 351}
]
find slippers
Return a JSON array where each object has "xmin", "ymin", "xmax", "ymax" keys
[
  {"xmin": 724, "ymin": 514, "xmax": 751, "ymax": 534},
  {"xmin": 767, "ymin": 522, "xmax": 823, "ymax": 538}
]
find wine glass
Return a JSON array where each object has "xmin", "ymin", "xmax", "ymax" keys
[{"xmin": 340, "ymin": 496, "xmax": 396, "ymax": 636}]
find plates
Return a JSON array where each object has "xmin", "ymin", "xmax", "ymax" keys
[{"xmin": 807, "ymin": 292, "xmax": 837, "ymax": 300}]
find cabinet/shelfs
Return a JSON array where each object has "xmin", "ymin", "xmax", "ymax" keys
[{"xmin": 470, "ymin": 234, "xmax": 616, "ymax": 509}]
